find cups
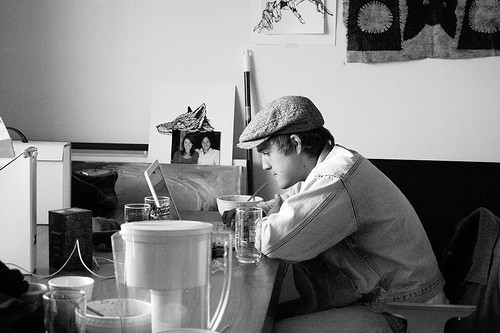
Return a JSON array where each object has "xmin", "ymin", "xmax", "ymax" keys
[
  {"xmin": 124, "ymin": 203, "xmax": 152, "ymax": 223},
  {"xmin": 145, "ymin": 196, "xmax": 170, "ymax": 220},
  {"xmin": 42, "ymin": 289, "xmax": 87, "ymax": 333},
  {"xmin": 234, "ymin": 206, "xmax": 263, "ymax": 264},
  {"xmin": 148, "ymin": 288, "xmax": 182, "ymax": 333},
  {"xmin": 18, "ymin": 282, "xmax": 48, "ymax": 310},
  {"xmin": 47, "ymin": 275, "xmax": 95, "ymax": 302}
]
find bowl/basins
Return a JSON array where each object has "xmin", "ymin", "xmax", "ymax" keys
[{"xmin": 215, "ymin": 195, "xmax": 264, "ymax": 221}]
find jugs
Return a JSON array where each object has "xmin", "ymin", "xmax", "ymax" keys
[{"xmin": 111, "ymin": 221, "xmax": 233, "ymax": 333}]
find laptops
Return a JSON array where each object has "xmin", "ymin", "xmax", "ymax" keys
[{"xmin": 144, "ymin": 160, "xmax": 247, "ymax": 249}]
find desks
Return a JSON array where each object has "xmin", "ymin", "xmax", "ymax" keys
[{"xmin": 24, "ymin": 210, "xmax": 288, "ymax": 333}]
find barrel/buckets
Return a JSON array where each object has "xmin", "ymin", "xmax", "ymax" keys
[{"xmin": 74, "ymin": 297, "xmax": 152, "ymax": 333}]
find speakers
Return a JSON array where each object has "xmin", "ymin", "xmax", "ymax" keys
[{"xmin": 48, "ymin": 208, "xmax": 93, "ymax": 270}]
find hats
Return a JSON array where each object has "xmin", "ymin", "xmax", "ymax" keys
[{"xmin": 237, "ymin": 96, "xmax": 324, "ymax": 150}]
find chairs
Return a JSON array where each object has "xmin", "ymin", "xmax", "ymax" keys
[{"xmin": 375, "ymin": 206, "xmax": 500, "ymax": 332}]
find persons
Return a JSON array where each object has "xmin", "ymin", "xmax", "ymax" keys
[
  {"xmin": 222, "ymin": 96, "xmax": 448, "ymax": 333},
  {"xmin": 194, "ymin": 136, "xmax": 220, "ymax": 165},
  {"xmin": 172, "ymin": 134, "xmax": 199, "ymax": 164}
]
